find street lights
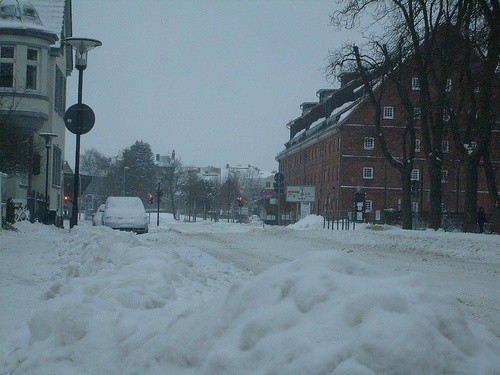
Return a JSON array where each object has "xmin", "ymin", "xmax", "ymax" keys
[
  {"xmin": 124, "ymin": 167, "xmax": 129, "ymax": 196},
  {"xmin": 64, "ymin": 36, "xmax": 103, "ymax": 229},
  {"xmin": 38, "ymin": 132, "xmax": 58, "ymax": 225},
  {"xmin": 156, "ymin": 175, "xmax": 165, "ymax": 226}
]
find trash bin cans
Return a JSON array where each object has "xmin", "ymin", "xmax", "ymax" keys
[{"xmin": 48, "ymin": 209, "xmax": 58, "ymax": 225}]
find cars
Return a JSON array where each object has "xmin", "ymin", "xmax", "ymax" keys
[{"xmin": 92, "ymin": 197, "xmax": 149, "ymax": 234}]
